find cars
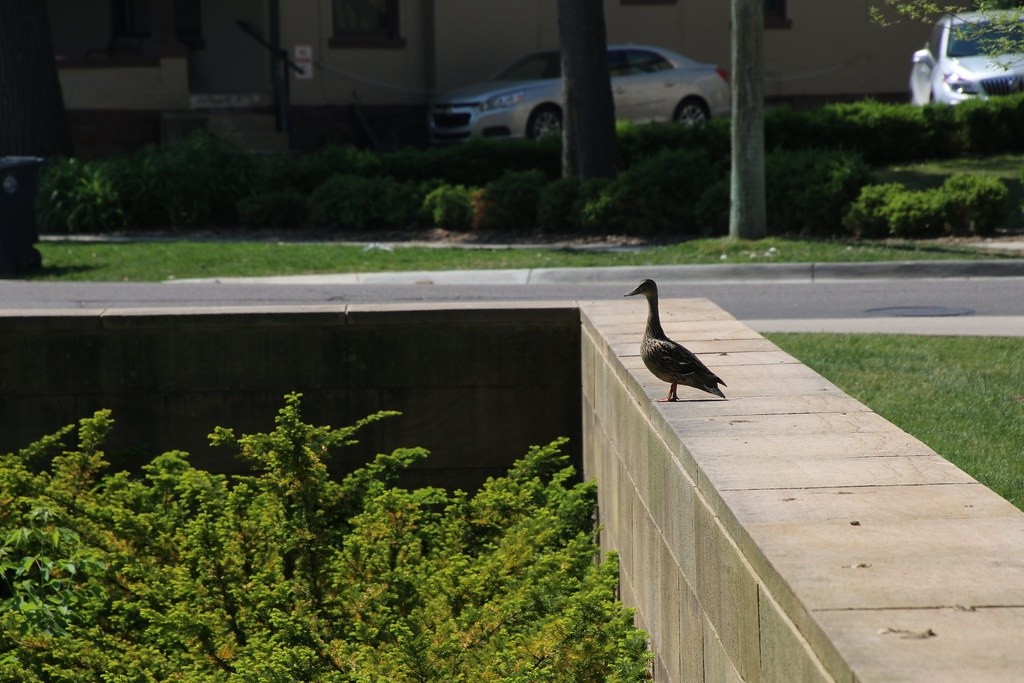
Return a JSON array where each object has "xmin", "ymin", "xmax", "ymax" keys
[
  {"xmin": 430, "ymin": 39, "xmax": 735, "ymax": 155},
  {"xmin": 907, "ymin": 10, "xmax": 1023, "ymax": 103}
]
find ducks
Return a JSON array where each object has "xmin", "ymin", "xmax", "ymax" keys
[{"xmin": 623, "ymin": 279, "xmax": 727, "ymax": 402}]
